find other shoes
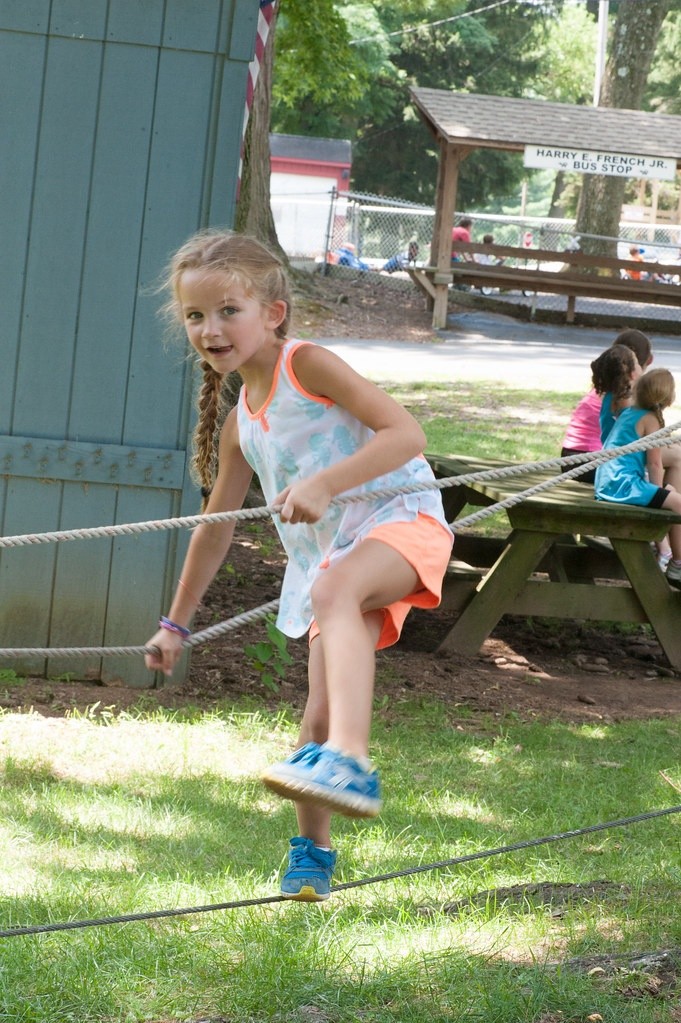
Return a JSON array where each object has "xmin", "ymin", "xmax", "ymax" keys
[
  {"xmin": 657, "ymin": 553, "xmax": 674, "ymax": 573},
  {"xmin": 666, "ymin": 559, "xmax": 681, "ymax": 580}
]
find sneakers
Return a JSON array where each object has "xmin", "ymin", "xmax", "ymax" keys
[
  {"xmin": 262, "ymin": 744, "xmax": 382, "ymax": 818},
  {"xmin": 280, "ymin": 837, "xmax": 340, "ymax": 902}
]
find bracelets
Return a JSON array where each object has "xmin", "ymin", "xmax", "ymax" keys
[
  {"xmin": 178, "ymin": 579, "xmax": 201, "ymax": 605},
  {"xmin": 159, "ymin": 616, "xmax": 190, "ymax": 638}
]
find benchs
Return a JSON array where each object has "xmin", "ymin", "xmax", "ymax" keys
[
  {"xmin": 440, "ymin": 552, "xmax": 480, "ymax": 582},
  {"xmin": 579, "ymin": 532, "xmax": 613, "ymax": 554}
]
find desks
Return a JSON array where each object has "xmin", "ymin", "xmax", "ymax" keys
[{"xmin": 423, "ymin": 452, "xmax": 681, "ymax": 676}]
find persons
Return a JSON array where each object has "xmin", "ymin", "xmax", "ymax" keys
[
  {"xmin": 624, "ymin": 247, "xmax": 677, "ymax": 285},
  {"xmin": 561, "ymin": 329, "xmax": 681, "ymax": 591},
  {"xmin": 378, "ymin": 241, "xmax": 419, "ymax": 274},
  {"xmin": 145, "ymin": 230, "xmax": 454, "ymax": 902},
  {"xmin": 336, "ymin": 243, "xmax": 369, "ymax": 270},
  {"xmin": 451, "ymin": 219, "xmax": 506, "ymax": 267}
]
